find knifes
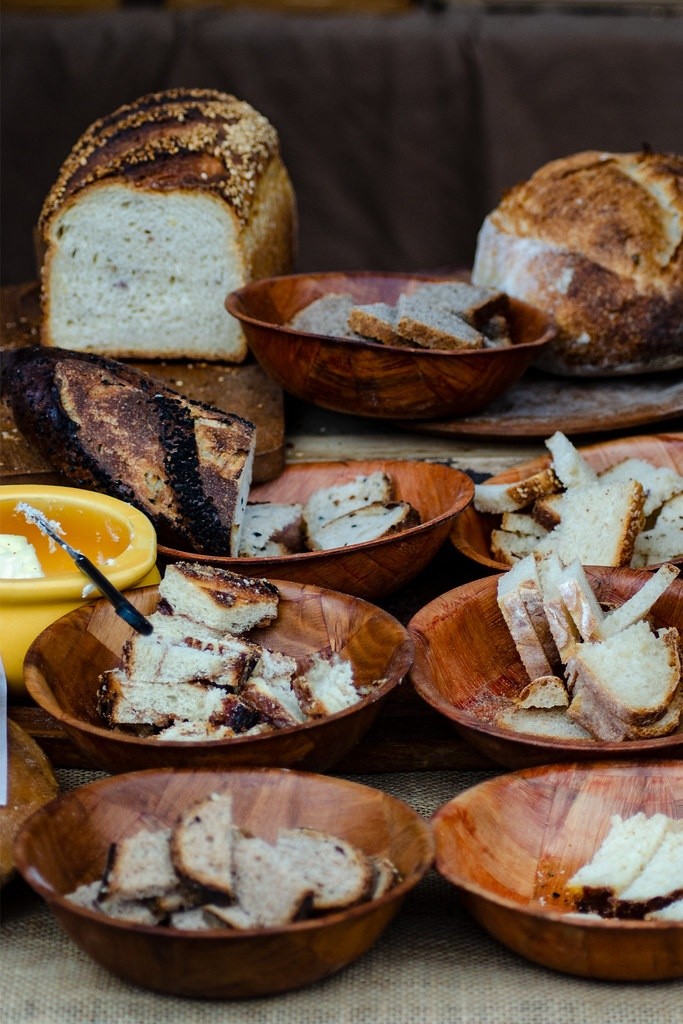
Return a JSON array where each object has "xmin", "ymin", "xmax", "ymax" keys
[{"xmin": 21, "ymin": 505, "xmax": 154, "ymax": 637}]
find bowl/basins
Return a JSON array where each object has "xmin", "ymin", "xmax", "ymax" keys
[
  {"xmin": 448, "ymin": 432, "xmax": 683, "ymax": 571},
  {"xmin": 0, "ymin": 483, "xmax": 161, "ymax": 692},
  {"xmin": 226, "ymin": 272, "xmax": 557, "ymax": 421},
  {"xmin": 158, "ymin": 462, "xmax": 476, "ymax": 604},
  {"xmin": 428, "ymin": 760, "xmax": 683, "ymax": 984},
  {"xmin": 11, "ymin": 765, "xmax": 438, "ymax": 1003},
  {"xmin": 406, "ymin": 569, "xmax": 683, "ymax": 760},
  {"xmin": 21, "ymin": 578, "xmax": 416, "ymax": 775}
]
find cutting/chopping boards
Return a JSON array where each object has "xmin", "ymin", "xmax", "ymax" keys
[{"xmin": 0, "ymin": 281, "xmax": 285, "ymax": 490}]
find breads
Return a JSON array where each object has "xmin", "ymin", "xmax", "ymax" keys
[
  {"xmin": 471, "ymin": 151, "xmax": 683, "ymax": 373},
  {"xmin": 0, "ymin": 344, "xmax": 683, "ymax": 929},
  {"xmin": 37, "ymin": 88, "xmax": 296, "ymax": 364},
  {"xmin": 284, "ymin": 282, "xmax": 509, "ymax": 351}
]
